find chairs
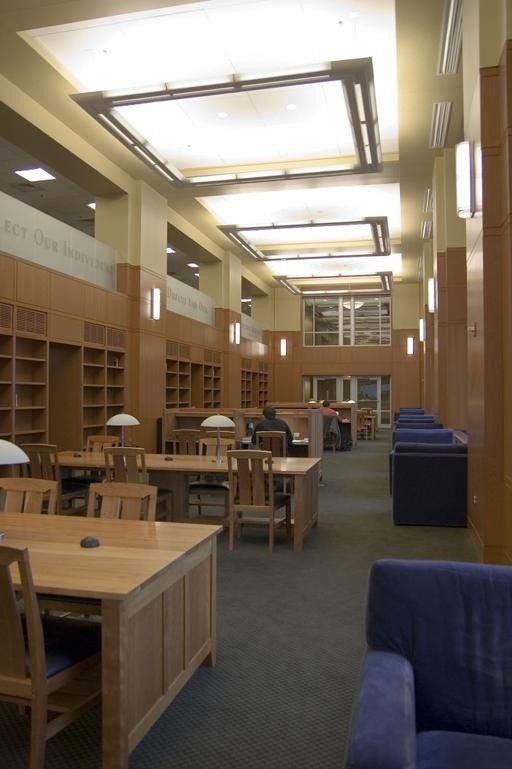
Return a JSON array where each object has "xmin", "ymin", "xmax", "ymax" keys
[
  {"xmin": 389, "ymin": 406, "xmax": 467, "ymax": 527},
  {"xmin": 346, "ymin": 559, "xmax": 512, "ymax": 767}
]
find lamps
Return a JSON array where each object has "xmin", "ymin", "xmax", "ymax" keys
[
  {"xmin": 343, "ymin": 301, "xmax": 365, "ymax": 309},
  {"xmin": 281, "ymin": 337, "xmax": 287, "ymax": 356},
  {"xmin": 419, "ymin": 318, "xmax": 426, "ymax": 341},
  {"xmin": 427, "ymin": 277, "xmax": 436, "ymax": 312},
  {"xmin": 407, "ymin": 335, "xmax": 414, "ymax": 354},
  {"xmin": 234, "ymin": 322, "xmax": 241, "ymax": 344},
  {"xmin": 151, "ymin": 286, "xmax": 161, "ymax": 320},
  {"xmin": 454, "ymin": 138, "xmax": 475, "ymax": 218}
]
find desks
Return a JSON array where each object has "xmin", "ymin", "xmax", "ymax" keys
[{"xmin": 265, "ymin": 401, "xmax": 357, "ymax": 449}]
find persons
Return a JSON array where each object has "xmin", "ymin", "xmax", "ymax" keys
[
  {"xmin": 319, "ymin": 400, "xmax": 345, "ymax": 423},
  {"xmin": 251, "ymin": 405, "xmax": 293, "ymax": 457}
]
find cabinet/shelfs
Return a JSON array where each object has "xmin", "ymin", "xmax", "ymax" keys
[
  {"xmin": 0, "ymin": 298, "xmax": 125, "ymax": 452},
  {"xmin": 241, "ymin": 356, "xmax": 269, "ymax": 407},
  {"xmin": 166, "ymin": 338, "xmax": 222, "ymax": 408}
]
